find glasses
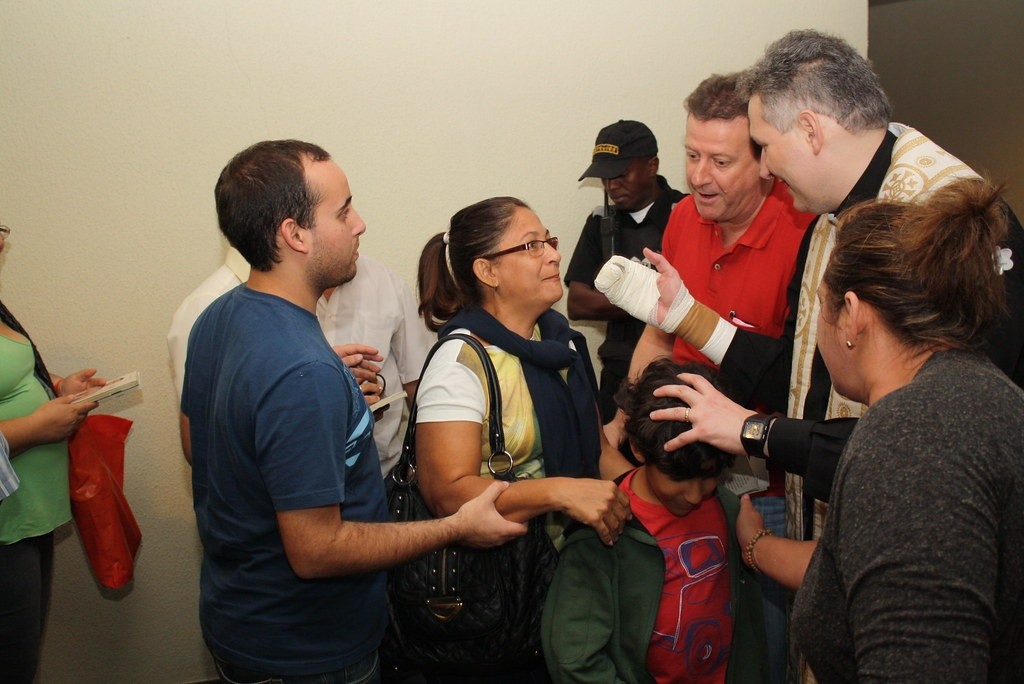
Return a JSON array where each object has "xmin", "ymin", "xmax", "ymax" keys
[
  {"xmin": 0, "ymin": 225, "xmax": 10, "ymax": 238},
  {"xmin": 483, "ymin": 237, "xmax": 558, "ymax": 259}
]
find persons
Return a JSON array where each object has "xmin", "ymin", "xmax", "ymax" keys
[
  {"xmin": 76, "ymin": 378, "xmax": 124, "ymax": 399},
  {"xmin": 563, "ymin": 119, "xmax": 690, "ymax": 428},
  {"xmin": 416, "ymin": 197, "xmax": 634, "ymax": 684},
  {"xmin": 600, "ymin": 72, "xmax": 818, "ymax": 684},
  {"xmin": 736, "ymin": 180, "xmax": 1024, "ymax": 684},
  {"xmin": 594, "ymin": 30, "xmax": 1024, "ymax": 684},
  {"xmin": 180, "ymin": 140, "xmax": 529, "ymax": 684},
  {"xmin": 542, "ymin": 359, "xmax": 745, "ymax": 684},
  {"xmin": 166, "ymin": 244, "xmax": 436, "ymax": 484},
  {"xmin": 0, "ymin": 226, "xmax": 106, "ymax": 684}
]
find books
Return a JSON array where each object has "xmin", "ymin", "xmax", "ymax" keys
[{"xmin": 71, "ymin": 371, "xmax": 143, "ymax": 404}]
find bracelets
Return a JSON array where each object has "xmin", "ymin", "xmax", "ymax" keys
[
  {"xmin": 744, "ymin": 529, "xmax": 772, "ymax": 572},
  {"xmin": 54, "ymin": 379, "xmax": 62, "ymax": 394}
]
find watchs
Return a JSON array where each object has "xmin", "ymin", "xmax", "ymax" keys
[{"xmin": 740, "ymin": 412, "xmax": 778, "ymax": 459}]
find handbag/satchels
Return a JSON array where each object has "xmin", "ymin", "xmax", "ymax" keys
[
  {"xmin": 381, "ymin": 334, "xmax": 559, "ymax": 670},
  {"xmin": 55, "ymin": 378, "xmax": 142, "ymax": 590}
]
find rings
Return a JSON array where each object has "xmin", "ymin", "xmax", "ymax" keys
[{"xmin": 685, "ymin": 408, "xmax": 690, "ymax": 422}]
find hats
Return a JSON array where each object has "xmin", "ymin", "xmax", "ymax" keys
[{"xmin": 579, "ymin": 119, "xmax": 658, "ymax": 181}]
